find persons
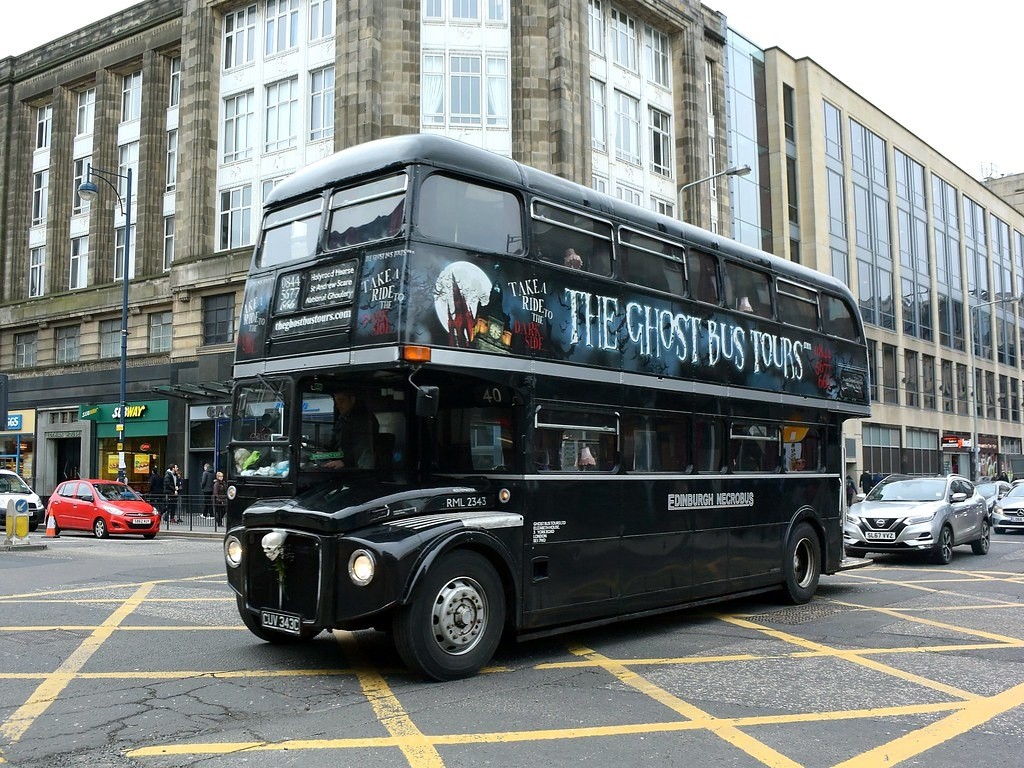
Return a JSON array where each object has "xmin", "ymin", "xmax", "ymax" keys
[
  {"xmin": 198, "ymin": 463, "xmax": 217, "ymax": 520},
  {"xmin": 163, "ymin": 463, "xmax": 178, "ymax": 525},
  {"xmin": 309, "ymin": 385, "xmax": 385, "ymax": 471},
  {"xmin": 989, "ymin": 471, "xmax": 1010, "ymax": 483},
  {"xmin": 859, "ymin": 468, "xmax": 875, "ymax": 497},
  {"xmin": 162, "ymin": 470, "xmax": 184, "ymax": 523},
  {"xmin": 212, "ymin": 471, "xmax": 229, "ymax": 527},
  {"xmin": 147, "ymin": 467, "xmax": 166, "ymax": 518},
  {"xmin": 846, "ymin": 475, "xmax": 859, "ymax": 509},
  {"xmin": 873, "ymin": 471, "xmax": 885, "ymax": 486}
]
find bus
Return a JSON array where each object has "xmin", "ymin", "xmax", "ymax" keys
[{"xmin": 225, "ymin": 132, "xmax": 872, "ymax": 682}]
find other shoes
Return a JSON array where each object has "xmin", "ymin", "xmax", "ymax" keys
[
  {"xmin": 200, "ymin": 514, "xmax": 206, "ymax": 519},
  {"xmin": 209, "ymin": 517, "xmax": 214, "ymax": 519},
  {"xmin": 218, "ymin": 524, "xmax": 224, "ymax": 527},
  {"xmin": 170, "ymin": 518, "xmax": 179, "ymax": 523},
  {"xmin": 177, "ymin": 519, "xmax": 183, "ymax": 522}
]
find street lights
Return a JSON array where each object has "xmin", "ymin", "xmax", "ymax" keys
[
  {"xmin": 679, "ymin": 163, "xmax": 751, "ymax": 222},
  {"xmin": 78, "ymin": 163, "xmax": 128, "ymax": 486},
  {"xmin": 970, "ymin": 296, "xmax": 1021, "ymax": 481}
]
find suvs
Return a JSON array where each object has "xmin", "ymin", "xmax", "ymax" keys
[
  {"xmin": 843, "ymin": 472, "xmax": 990, "ymax": 564},
  {"xmin": 0, "ymin": 469, "xmax": 46, "ymax": 533}
]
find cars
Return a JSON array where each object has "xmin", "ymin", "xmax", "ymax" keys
[
  {"xmin": 45, "ymin": 480, "xmax": 162, "ymax": 539},
  {"xmin": 991, "ymin": 483, "xmax": 1024, "ymax": 533},
  {"xmin": 972, "ymin": 481, "xmax": 1013, "ymax": 526}
]
